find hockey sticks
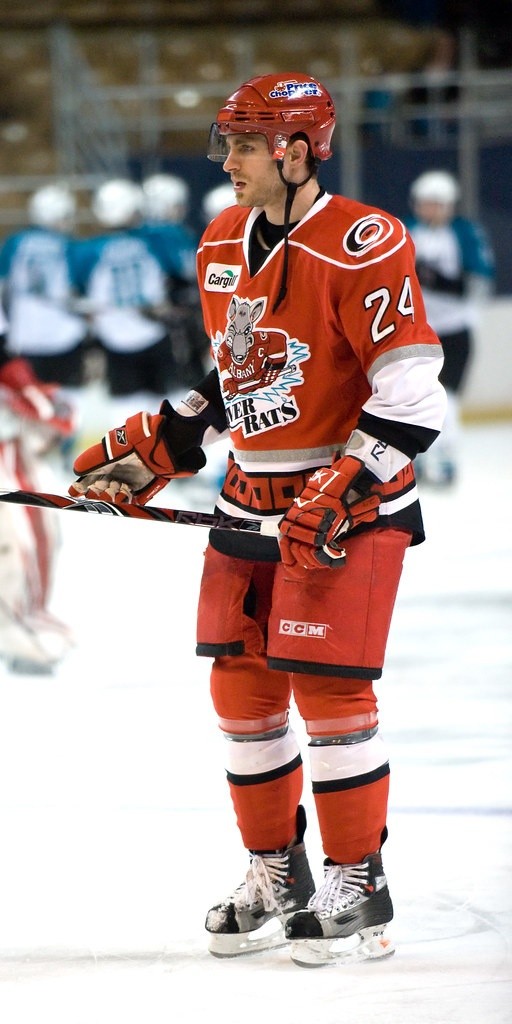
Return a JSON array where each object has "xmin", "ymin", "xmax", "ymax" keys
[{"xmin": 0, "ymin": 479, "xmax": 282, "ymax": 540}]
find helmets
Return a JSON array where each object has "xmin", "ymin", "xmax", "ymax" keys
[
  {"xmin": 25, "ymin": 185, "xmax": 76, "ymax": 226},
  {"xmin": 411, "ymin": 169, "xmax": 460, "ymax": 201},
  {"xmin": 214, "ymin": 74, "xmax": 337, "ymax": 163},
  {"xmin": 138, "ymin": 174, "xmax": 190, "ymax": 219},
  {"xmin": 91, "ymin": 179, "xmax": 141, "ymax": 225},
  {"xmin": 203, "ymin": 183, "xmax": 239, "ymax": 223}
]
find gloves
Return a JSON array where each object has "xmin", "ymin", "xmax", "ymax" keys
[
  {"xmin": 2, "ymin": 358, "xmax": 79, "ymax": 453},
  {"xmin": 70, "ymin": 412, "xmax": 208, "ymax": 507},
  {"xmin": 275, "ymin": 449, "xmax": 380, "ymax": 579}
]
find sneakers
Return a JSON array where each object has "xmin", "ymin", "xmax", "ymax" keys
[
  {"xmin": 285, "ymin": 824, "xmax": 398, "ymax": 963},
  {"xmin": 205, "ymin": 804, "xmax": 318, "ymax": 955}
]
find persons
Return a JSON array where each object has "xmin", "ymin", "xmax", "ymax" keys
[
  {"xmin": 0, "ymin": 308, "xmax": 72, "ymax": 669},
  {"xmin": 1, "ymin": 169, "xmax": 491, "ymax": 489},
  {"xmin": 67, "ymin": 73, "xmax": 447, "ymax": 940}
]
